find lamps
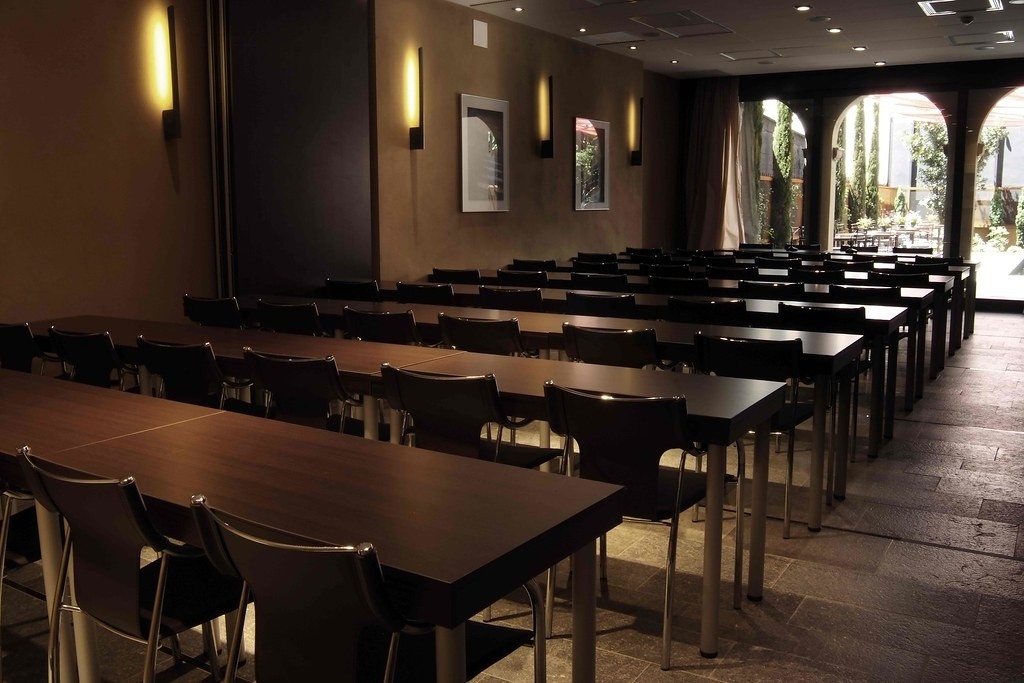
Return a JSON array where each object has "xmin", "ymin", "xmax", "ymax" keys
[{"xmin": 161, "ymin": 5, "xmax": 182, "ymax": 140}]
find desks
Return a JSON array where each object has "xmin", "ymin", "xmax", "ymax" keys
[
  {"xmin": 548, "ymin": 317, "xmax": 865, "ymax": 532},
  {"xmin": 0, "ymin": 242, "xmax": 964, "ymax": 683},
  {"xmin": 24, "ymin": 315, "xmax": 467, "ymax": 442},
  {"xmin": 235, "ymin": 293, "xmax": 566, "ymax": 360},
  {"xmin": 556, "ymin": 260, "xmax": 641, "ymax": 274},
  {"xmin": 370, "ymin": 352, "xmax": 788, "ymax": 660},
  {"xmin": 801, "ymin": 260, "xmax": 971, "ymax": 358},
  {"xmin": 702, "ymin": 296, "xmax": 909, "ymax": 459},
  {"xmin": 757, "ymin": 267, "xmax": 956, "ymax": 381},
  {"xmin": 377, "ymin": 280, "xmax": 704, "ymax": 320},
  {"xmin": 466, "ymin": 268, "xmax": 648, "ymax": 292},
  {"xmin": 709, "ymin": 279, "xmax": 935, "ymax": 412},
  {"xmin": 831, "ymin": 255, "xmax": 981, "ymax": 335},
  {"xmin": 572, "ymin": 254, "xmax": 755, "ymax": 268},
  {"xmin": 29, "ymin": 411, "xmax": 624, "ymax": 683},
  {"xmin": 0, "ymin": 369, "xmax": 222, "ymax": 683}
]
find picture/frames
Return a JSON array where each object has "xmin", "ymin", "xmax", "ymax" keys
[
  {"xmin": 575, "ymin": 117, "xmax": 611, "ymax": 212},
  {"xmin": 630, "ymin": 97, "xmax": 644, "ymax": 166},
  {"xmin": 539, "ymin": 76, "xmax": 554, "ymax": 159},
  {"xmin": 409, "ymin": 46, "xmax": 425, "ymax": 151},
  {"xmin": 459, "ymin": 93, "xmax": 510, "ymax": 213}
]
